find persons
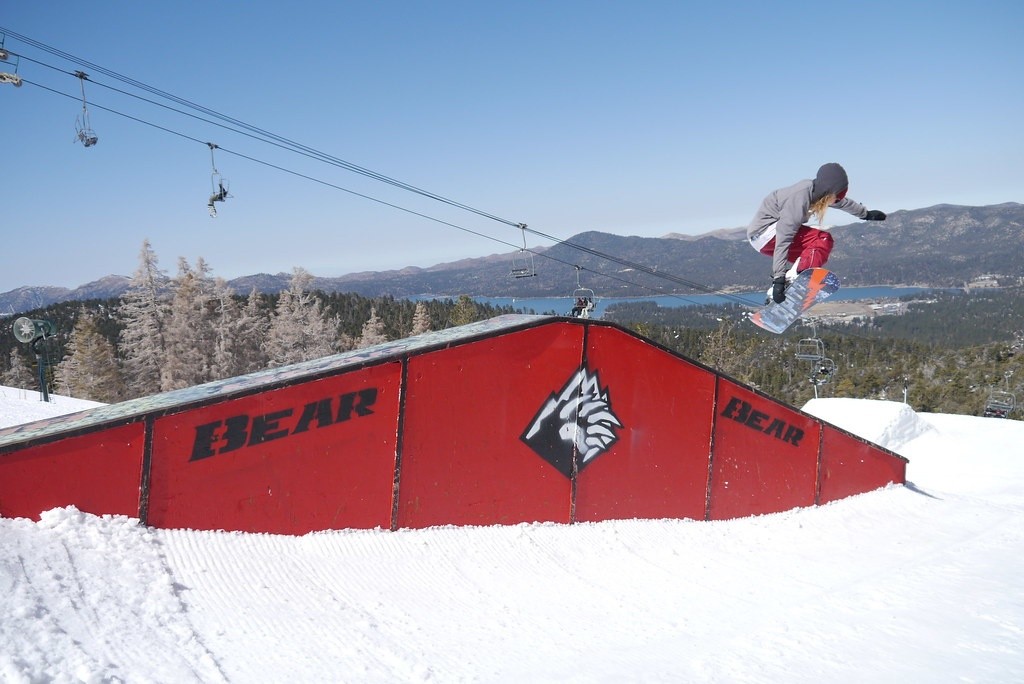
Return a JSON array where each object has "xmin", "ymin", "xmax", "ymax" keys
[{"xmin": 747, "ymin": 163, "xmax": 888, "ymax": 304}]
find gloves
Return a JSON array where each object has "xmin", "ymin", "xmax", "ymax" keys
[
  {"xmin": 860, "ymin": 210, "xmax": 886, "ymax": 220},
  {"xmin": 772, "ymin": 276, "xmax": 786, "ymax": 304}
]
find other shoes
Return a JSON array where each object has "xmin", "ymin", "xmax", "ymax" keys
[
  {"xmin": 786, "ymin": 257, "xmax": 801, "ymax": 284},
  {"xmin": 767, "ymin": 286, "xmax": 773, "ymax": 300}
]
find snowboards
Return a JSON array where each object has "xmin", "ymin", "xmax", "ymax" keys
[{"xmin": 748, "ymin": 266, "xmax": 842, "ymax": 335}]
